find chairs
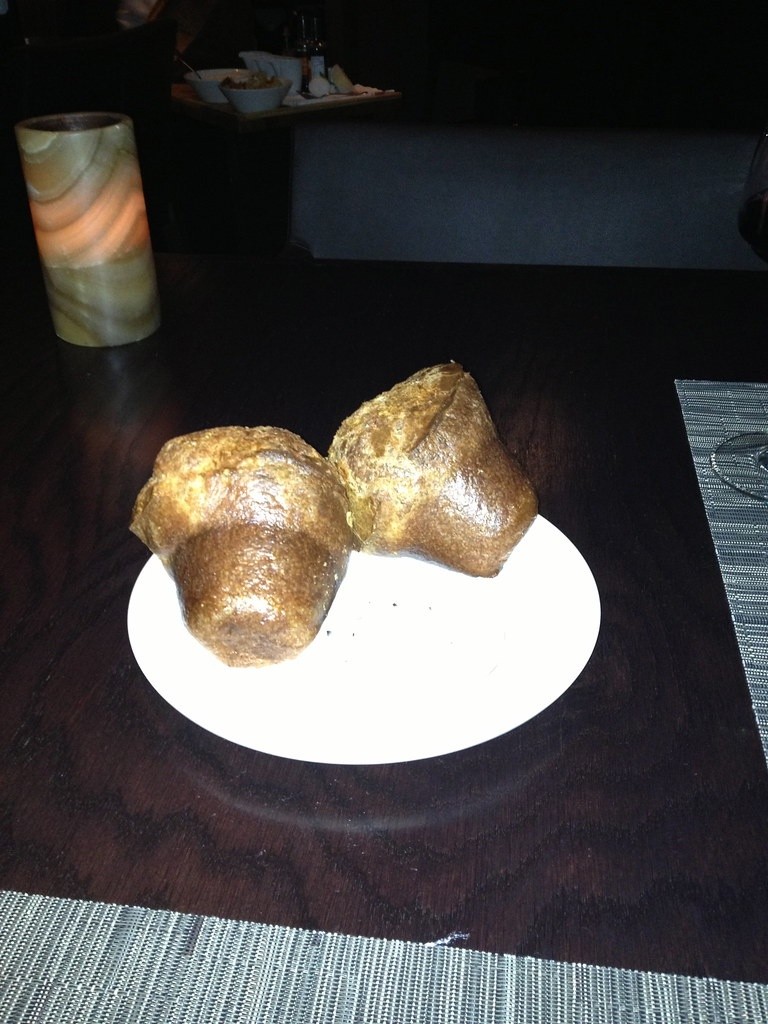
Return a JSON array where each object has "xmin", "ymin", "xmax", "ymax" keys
[{"xmin": 285, "ymin": 125, "xmax": 762, "ymax": 269}]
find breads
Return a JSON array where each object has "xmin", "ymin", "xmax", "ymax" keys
[
  {"xmin": 127, "ymin": 426, "xmax": 351, "ymax": 667},
  {"xmin": 327, "ymin": 361, "xmax": 538, "ymax": 579}
]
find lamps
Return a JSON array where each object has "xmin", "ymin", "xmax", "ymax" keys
[{"xmin": 15, "ymin": 110, "xmax": 164, "ymax": 348}]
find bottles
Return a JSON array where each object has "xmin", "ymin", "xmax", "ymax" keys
[
  {"xmin": 293, "ymin": 13, "xmax": 311, "ymax": 92},
  {"xmin": 307, "ymin": 11, "xmax": 328, "ymax": 93}
]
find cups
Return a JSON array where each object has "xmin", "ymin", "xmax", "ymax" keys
[
  {"xmin": 14, "ymin": 113, "xmax": 162, "ymax": 346},
  {"xmin": 239, "ymin": 50, "xmax": 301, "ymax": 94}
]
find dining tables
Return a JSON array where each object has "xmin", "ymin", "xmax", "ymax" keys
[
  {"xmin": 1, "ymin": 255, "xmax": 768, "ymax": 1024},
  {"xmin": 177, "ymin": 79, "xmax": 401, "ymax": 121}
]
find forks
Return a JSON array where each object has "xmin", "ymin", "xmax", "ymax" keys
[{"xmin": 296, "ymin": 89, "xmax": 360, "ymax": 100}]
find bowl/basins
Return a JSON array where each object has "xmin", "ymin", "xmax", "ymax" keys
[
  {"xmin": 183, "ymin": 67, "xmax": 253, "ymax": 103},
  {"xmin": 218, "ymin": 75, "xmax": 292, "ymax": 113}
]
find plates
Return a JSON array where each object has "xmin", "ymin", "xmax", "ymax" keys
[{"xmin": 127, "ymin": 512, "xmax": 604, "ymax": 766}]
